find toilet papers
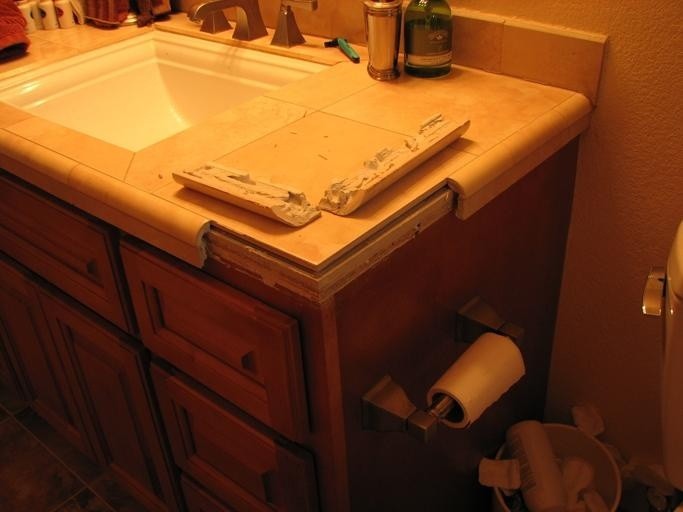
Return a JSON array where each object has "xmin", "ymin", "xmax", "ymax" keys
[{"xmin": 426, "ymin": 332, "xmax": 526, "ymax": 430}]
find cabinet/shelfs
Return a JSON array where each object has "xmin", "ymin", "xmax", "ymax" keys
[
  {"xmin": 117, "ymin": 131, "xmax": 578, "ymax": 509},
  {"xmin": 0, "ymin": 168, "xmax": 182, "ymax": 510}
]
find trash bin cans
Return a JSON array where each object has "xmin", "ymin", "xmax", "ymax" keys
[{"xmin": 492, "ymin": 423, "xmax": 623, "ymax": 512}]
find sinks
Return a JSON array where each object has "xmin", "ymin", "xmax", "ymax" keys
[{"xmin": 0, "ymin": 23, "xmax": 341, "ymax": 153}]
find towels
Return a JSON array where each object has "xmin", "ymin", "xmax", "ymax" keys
[{"xmin": 0, "ymin": 0, "xmax": 31, "ymax": 59}]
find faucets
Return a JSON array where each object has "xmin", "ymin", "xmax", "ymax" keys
[{"xmin": 189, "ymin": 0, "xmax": 269, "ymax": 41}]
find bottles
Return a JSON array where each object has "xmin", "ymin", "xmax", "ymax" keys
[
  {"xmin": 16, "ymin": 1, "xmax": 75, "ymax": 31},
  {"xmin": 404, "ymin": 0, "xmax": 453, "ymax": 77},
  {"xmin": 508, "ymin": 417, "xmax": 568, "ymax": 510},
  {"xmin": 362, "ymin": 0, "xmax": 402, "ymax": 82}
]
